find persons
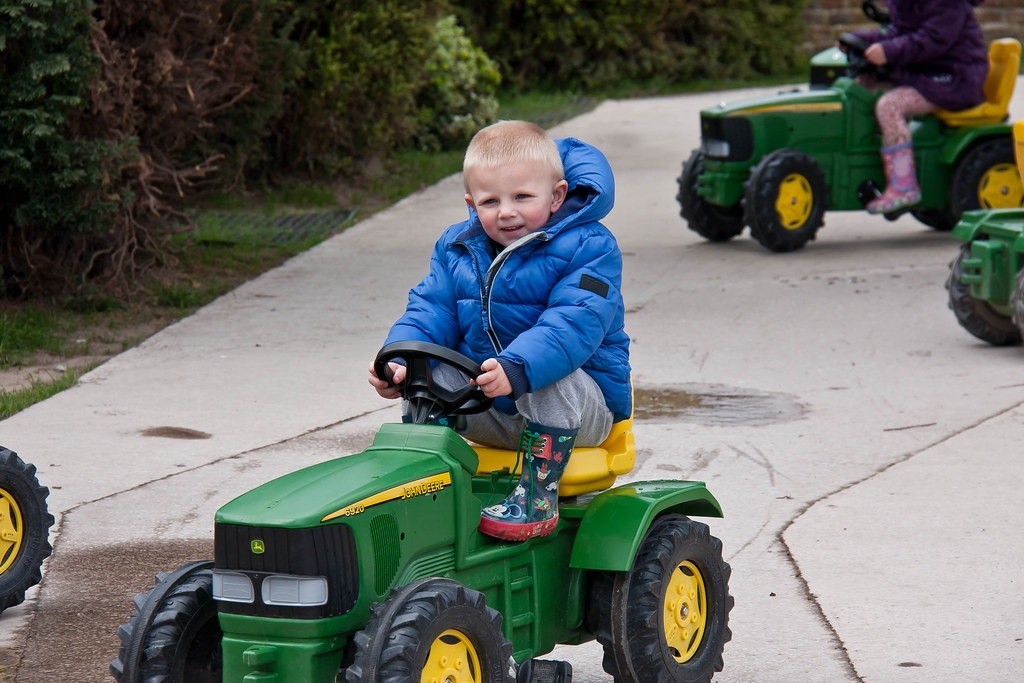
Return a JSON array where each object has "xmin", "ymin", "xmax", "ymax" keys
[
  {"xmin": 850, "ymin": 0, "xmax": 990, "ymax": 215},
  {"xmin": 367, "ymin": 120, "xmax": 633, "ymax": 543}
]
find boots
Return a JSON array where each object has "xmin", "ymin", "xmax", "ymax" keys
[
  {"xmin": 477, "ymin": 419, "xmax": 579, "ymax": 541},
  {"xmin": 867, "ymin": 140, "xmax": 920, "ymax": 214}
]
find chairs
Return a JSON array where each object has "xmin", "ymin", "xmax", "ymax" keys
[
  {"xmin": 932, "ymin": 37, "xmax": 1021, "ymax": 128},
  {"xmin": 467, "ymin": 370, "xmax": 635, "ymax": 497}
]
